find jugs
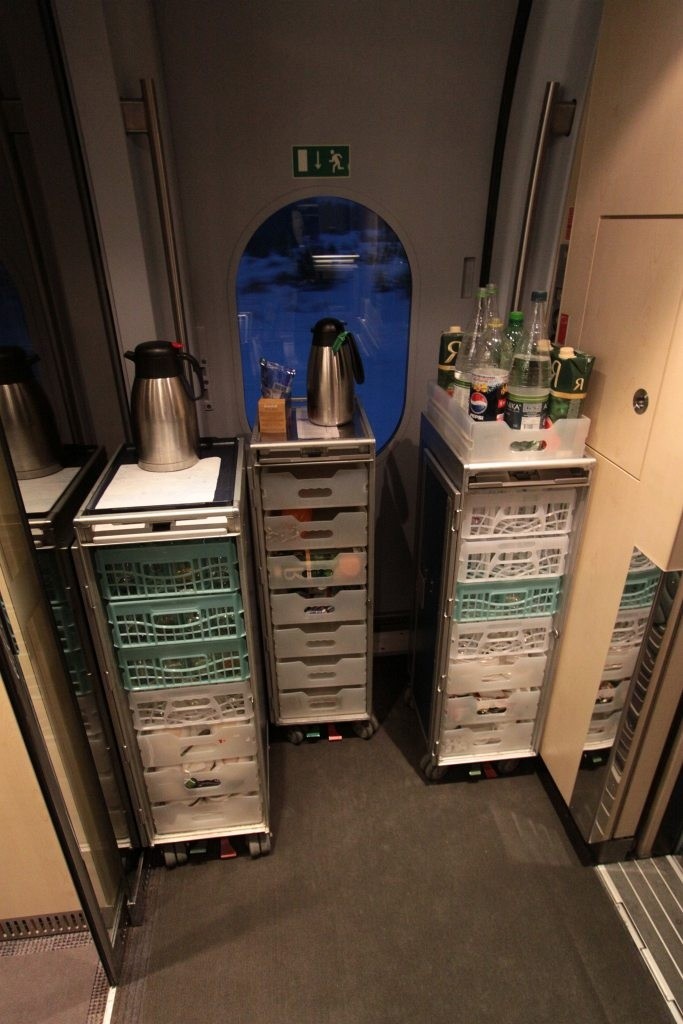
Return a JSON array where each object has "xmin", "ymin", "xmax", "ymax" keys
[
  {"xmin": 123, "ymin": 342, "xmax": 206, "ymax": 473},
  {"xmin": 305, "ymin": 318, "xmax": 366, "ymax": 426}
]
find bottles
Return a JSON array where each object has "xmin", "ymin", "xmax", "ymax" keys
[{"xmin": 452, "ymin": 284, "xmax": 554, "ymax": 449}]
